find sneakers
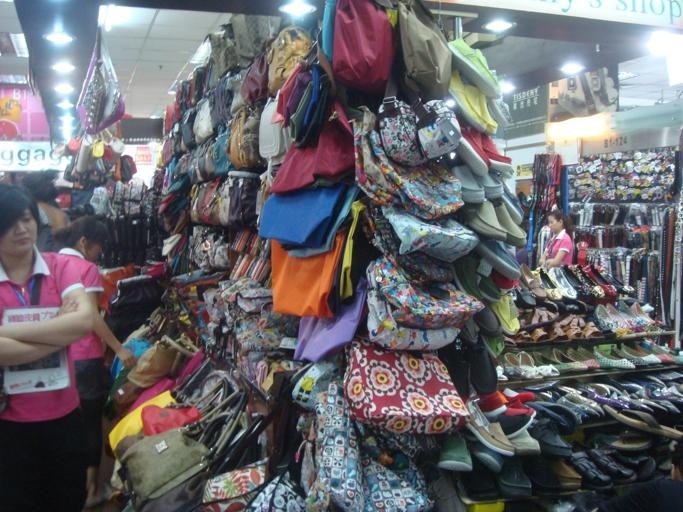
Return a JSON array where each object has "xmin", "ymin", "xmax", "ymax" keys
[{"xmin": 520, "ymin": 263, "xmax": 681, "ymax": 490}]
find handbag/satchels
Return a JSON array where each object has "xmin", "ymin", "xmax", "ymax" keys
[
  {"xmin": 64, "ymin": 69, "xmax": 122, "ymax": 187},
  {"xmin": 109, "ymin": 1, "xmax": 479, "ymax": 512}
]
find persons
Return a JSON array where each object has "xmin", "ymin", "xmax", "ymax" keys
[
  {"xmin": 0, "ymin": 182, "xmax": 93, "ymax": 512},
  {"xmin": 44, "ymin": 215, "xmax": 138, "ymax": 500},
  {"xmin": 20, "ymin": 169, "xmax": 72, "ymax": 233},
  {"xmin": 539, "ymin": 208, "xmax": 574, "ymax": 266}
]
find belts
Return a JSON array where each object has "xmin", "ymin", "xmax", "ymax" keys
[{"xmin": 569, "ymin": 204, "xmax": 683, "ymax": 348}]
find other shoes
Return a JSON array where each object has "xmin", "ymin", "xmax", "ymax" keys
[{"xmin": 449, "ymin": 39, "xmax": 539, "ymax": 497}]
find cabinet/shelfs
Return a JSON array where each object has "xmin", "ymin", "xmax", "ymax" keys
[{"xmin": 494, "ymin": 331, "xmax": 683, "ymax": 512}]
